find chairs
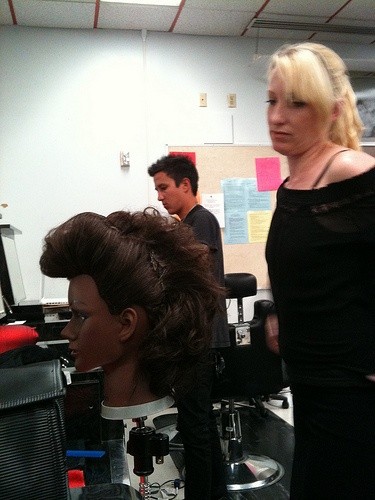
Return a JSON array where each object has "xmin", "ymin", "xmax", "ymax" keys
[{"xmin": 210, "ymin": 271, "xmax": 292, "ymax": 493}]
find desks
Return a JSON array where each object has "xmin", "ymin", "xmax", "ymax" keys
[{"xmin": 0, "ymin": 304, "xmax": 229, "ymax": 500}]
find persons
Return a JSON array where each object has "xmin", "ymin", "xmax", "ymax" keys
[
  {"xmin": 147, "ymin": 152, "xmax": 239, "ymax": 500},
  {"xmin": 260, "ymin": 38, "xmax": 375, "ymax": 500},
  {"xmin": 37, "ymin": 206, "xmax": 210, "ymax": 423}
]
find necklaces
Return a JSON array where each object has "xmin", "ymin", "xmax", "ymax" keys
[{"xmin": 179, "ymin": 203, "xmax": 200, "ymax": 226}]
